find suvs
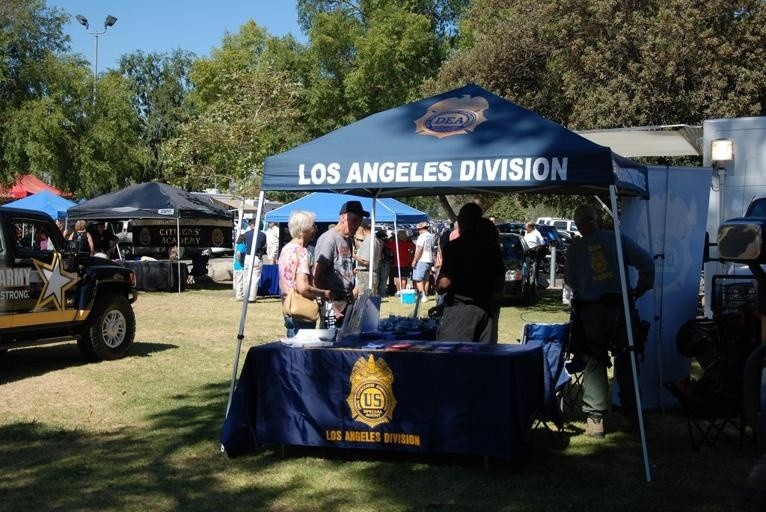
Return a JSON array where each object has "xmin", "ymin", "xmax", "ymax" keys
[{"xmin": 0, "ymin": 207, "xmax": 137, "ymax": 362}]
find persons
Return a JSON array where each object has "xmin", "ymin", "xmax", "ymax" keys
[
  {"xmin": 243, "ymin": 218, "xmax": 266, "ymax": 303},
  {"xmin": 313, "ymin": 201, "xmax": 369, "ymax": 329},
  {"xmin": 352, "ymin": 221, "xmax": 384, "ymax": 299},
  {"xmin": 376, "ymin": 230, "xmax": 391, "ymax": 302},
  {"xmin": 68, "ymin": 219, "xmax": 94, "ymax": 256},
  {"xmin": 523, "ymin": 222, "xmax": 545, "ymax": 250},
  {"xmin": 411, "ymin": 222, "xmax": 435, "ymax": 303},
  {"xmin": 266, "ymin": 222, "xmax": 279, "ymax": 264},
  {"xmin": 92, "ymin": 223, "xmax": 119, "ymax": 260},
  {"xmin": 564, "ymin": 204, "xmax": 655, "ymax": 438},
  {"xmin": 279, "ymin": 210, "xmax": 332, "ymax": 338},
  {"xmin": 386, "ymin": 230, "xmax": 408, "ymax": 297},
  {"xmin": 233, "ymin": 234, "xmax": 247, "ymax": 301},
  {"xmin": 436, "ymin": 202, "xmax": 506, "ymax": 344}
]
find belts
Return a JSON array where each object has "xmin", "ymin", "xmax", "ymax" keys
[{"xmin": 360, "ymin": 269, "xmax": 368, "ymax": 271}]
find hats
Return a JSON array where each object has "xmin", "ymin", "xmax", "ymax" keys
[
  {"xmin": 416, "ymin": 221, "xmax": 430, "ymax": 229},
  {"xmin": 339, "ymin": 200, "xmax": 370, "ymax": 217},
  {"xmin": 574, "ymin": 204, "xmax": 599, "ymax": 225}
]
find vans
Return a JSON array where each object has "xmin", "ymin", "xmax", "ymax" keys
[
  {"xmin": 496, "ymin": 222, "xmax": 563, "ymax": 274},
  {"xmin": 536, "ymin": 217, "xmax": 583, "ymax": 241}
]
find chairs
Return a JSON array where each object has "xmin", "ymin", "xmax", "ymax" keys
[
  {"xmin": 560, "ymin": 361, "xmax": 589, "ymax": 411},
  {"xmin": 522, "ymin": 322, "xmax": 573, "ymax": 423}
]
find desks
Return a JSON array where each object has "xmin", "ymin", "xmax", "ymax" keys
[
  {"xmin": 256, "ymin": 261, "xmax": 280, "ymax": 297},
  {"xmin": 219, "ymin": 335, "xmax": 562, "ymax": 472},
  {"xmin": 118, "ymin": 260, "xmax": 188, "ymax": 294}
]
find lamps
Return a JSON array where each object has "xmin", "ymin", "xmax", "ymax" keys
[{"xmin": 710, "ymin": 137, "xmax": 735, "ymax": 170}]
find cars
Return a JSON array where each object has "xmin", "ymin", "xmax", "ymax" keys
[
  {"xmin": 496, "ymin": 233, "xmax": 539, "ymax": 305},
  {"xmin": 232, "ymin": 219, "xmax": 252, "ymax": 235},
  {"xmin": 200, "ymin": 237, "xmax": 235, "ymax": 257},
  {"xmin": 374, "ymin": 223, "xmax": 419, "ymax": 240}
]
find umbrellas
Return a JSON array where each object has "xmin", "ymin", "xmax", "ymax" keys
[
  {"xmin": 0, "ymin": 190, "xmax": 79, "ymax": 250},
  {"xmin": 0, "ymin": 175, "xmax": 73, "ymax": 202}
]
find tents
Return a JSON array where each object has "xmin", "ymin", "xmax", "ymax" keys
[
  {"xmin": 221, "ymin": 82, "xmax": 652, "ymax": 483},
  {"xmin": 67, "ymin": 181, "xmax": 235, "ymax": 295},
  {"xmin": 263, "ymin": 191, "xmax": 428, "ymax": 305}
]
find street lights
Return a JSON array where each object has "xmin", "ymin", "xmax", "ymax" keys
[
  {"xmin": 710, "ymin": 139, "xmax": 733, "ymax": 275},
  {"xmin": 76, "ymin": 14, "xmax": 118, "ymax": 120}
]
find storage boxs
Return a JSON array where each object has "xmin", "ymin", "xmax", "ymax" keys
[{"xmin": 399, "ymin": 285, "xmax": 417, "ymax": 304}]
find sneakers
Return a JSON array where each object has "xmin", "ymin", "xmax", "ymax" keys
[
  {"xmin": 420, "ymin": 297, "xmax": 427, "ymax": 302},
  {"xmin": 395, "ymin": 293, "xmax": 400, "ymax": 297},
  {"xmin": 621, "ymin": 413, "xmax": 636, "ymax": 432},
  {"xmin": 586, "ymin": 416, "xmax": 604, "ymax": 436}
]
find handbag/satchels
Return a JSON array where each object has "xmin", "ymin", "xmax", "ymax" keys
[{"xmin": 282, "ymin": 246, "xmax": 319, "ymax": 323}]
